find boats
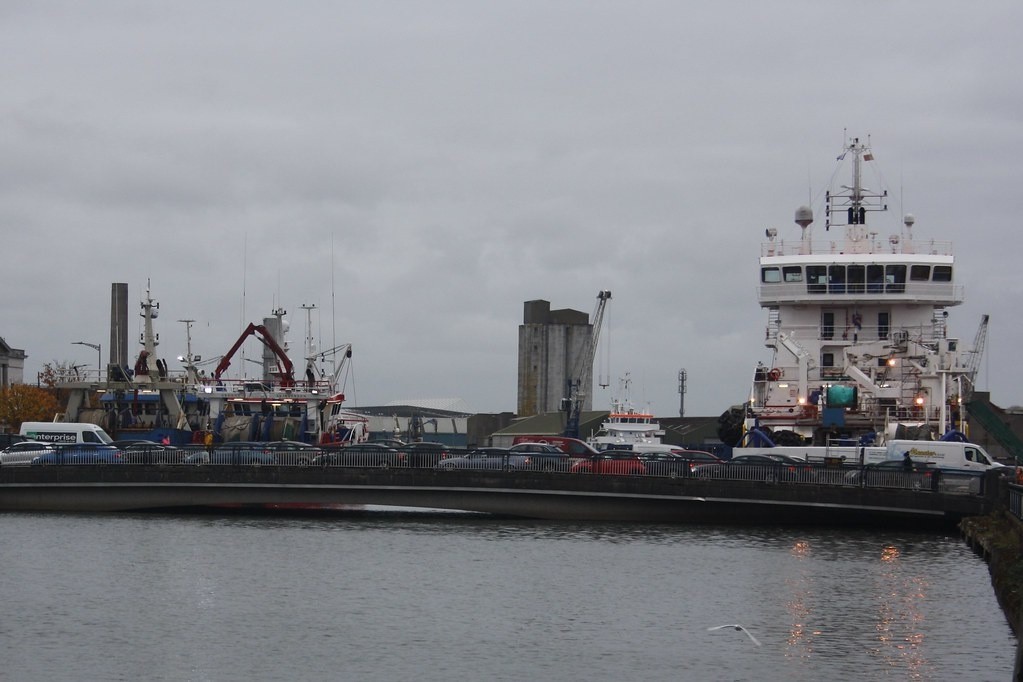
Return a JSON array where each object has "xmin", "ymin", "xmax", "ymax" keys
[
  {"xmin": 53, "ymin": 220, "xmax": 370, "ymax": 449},
  {"xmin": 585, "ymin": 370, "xmax": 663, "ymax": 453},
  {"xmin": 732, "ymin": 128, "xmax": 978, "ymax": 465}
]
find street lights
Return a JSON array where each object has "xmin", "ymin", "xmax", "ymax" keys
[
  {"xmin": 71, "ymin": 342, "xmax": 102, "ymax": 381},
  {"xmin": 177, "ymin": 319, "xmax": 197, "ymax": 389}
]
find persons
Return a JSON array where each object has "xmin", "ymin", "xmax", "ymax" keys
[{"xmin": 162, "ymin": 434, "xmax": 170, "ymax": 445}]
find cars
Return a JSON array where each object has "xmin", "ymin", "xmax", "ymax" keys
[
  {"xmin": 109, "ymin": 440, "xmax": 211, "ymax": 465},
  {"xmin": 436, "ymin": 443, "xmax": 570, "ymax": 470},
  {"xmin": 987, "ymin": 465, "xmax": 1023, "ymax": 485},
  {"xmin": 31, "ymin": 442, "xmax": 129, "ymax": 466},
  {"xmin": 689, "ymin": 454, "xmax": 820, "ymax": 482},
  {"xmin": 637, "ymin": 453, "xmax": 696, "ymax": 476},
  {"xmin": 670, "ymin": 450, "xmax": 726, "ymax": 468},
  {"xmin": 0, "ymin": 442, "xmax": 59, "ymax": 467},
  {"xmin": 210, "ymin": 440, "xmax": 325, "ymax": 467},
  {"xmin": 569, "ymin": 450, "xmax": 647, "ymax": 475},
  {"xmin": 313, "ymin": 440, "xmax": 452, "ymax": 468}
]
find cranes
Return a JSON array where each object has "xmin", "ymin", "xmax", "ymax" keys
[
  {"xmin": 962, "ymin": 314, "xmax": 990, "ymax": 393},
  {"xmin": 564, "ymin": 291, "xmax": 614, "ymax": 437}
]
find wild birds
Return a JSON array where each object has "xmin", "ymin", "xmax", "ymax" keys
[{"xmin": 707, "ymin": 624, "xmax": 761, "ymax": 646}]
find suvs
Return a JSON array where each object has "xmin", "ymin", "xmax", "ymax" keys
[
  {"xmin": 845, "ymin": 461, "xmax": 945, "ymax": 493},
  {"xmin": 0, "ymin": 433, "xmax": 28, "ymax": 451}
]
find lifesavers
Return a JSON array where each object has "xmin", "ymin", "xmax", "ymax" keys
[
  {"xmin": 770, "ymin": 368, "xmax": 782, "ymax": 380},
  {"xmin": 803, "ymin": 403, "xmax": 818, "ymax": 418}
]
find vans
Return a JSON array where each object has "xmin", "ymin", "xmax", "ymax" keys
[
  {"xmin": 632, "ymin": 443, "xmax": 683, "ymax": 454},
  {"xmin": 20, "ymin": 422, "xmax": 114, "ymax": 446},
  {"xmin": 885, "ymin": 439, "xmax": 1006, "ymax": 472}
]
